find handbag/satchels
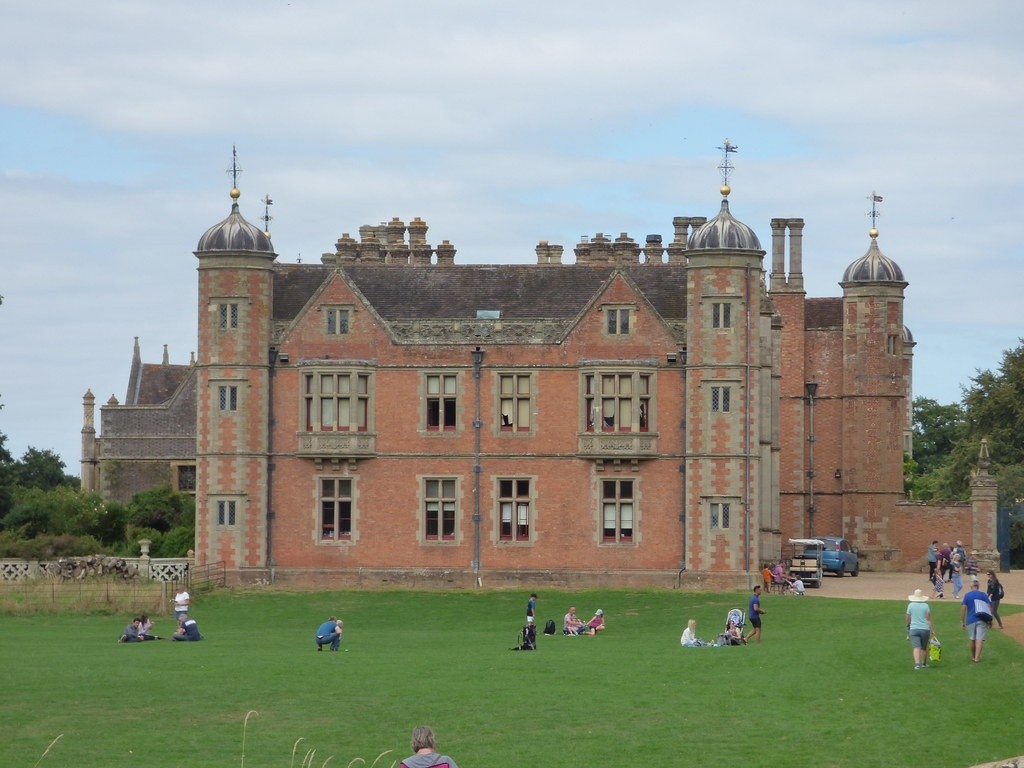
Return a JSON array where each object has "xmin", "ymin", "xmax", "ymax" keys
[
  {"xmin": 929, "ymin": 635, "xmax": 942, "ymax": 661},
  {"xmin": 974, "ymin": 592, "xmax": 993, "ymax": 622}
]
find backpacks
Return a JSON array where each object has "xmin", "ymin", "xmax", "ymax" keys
[
  {"xmin": 544, "ymin": 619, "xmax": 555, "ymax": 634},
  {"xmin": 996, "ymin": 581, "xmax": 1004, "ymax": 598}
]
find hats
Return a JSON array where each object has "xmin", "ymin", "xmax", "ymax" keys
[
  {"xmin": 530, "ymin": 592, "xmax": 537, "ymax": 598},
  {"xmin": 971, "ymin": 549, "xmax": 979, "ymax": 555},
  {"xmin": 595, "ymin": 609, "xmax": 603, "ymax": 616},
  {"xmin": 908, "ymin": 589, "xmax": 929, "ymax": 602}
]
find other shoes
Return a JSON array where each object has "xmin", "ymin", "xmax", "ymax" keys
[
  {"xmin": 914, "ymin": 664, "xmax": 920, "ymax": 669},
  {"xmin": 922, "ymin": 664, "xmax": 929, "ymax": 668}
]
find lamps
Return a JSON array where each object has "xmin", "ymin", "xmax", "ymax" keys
[
  {"xmin": 835, "ymin": 469, "xmax": 841, "ymax": 478},
  {"xmin": 278, "ymin": 352, "xmax": 289, "ymax": 363},
  {"xmin": 666, "ymin": 352, "xmax": 677, "ymax": 362}
]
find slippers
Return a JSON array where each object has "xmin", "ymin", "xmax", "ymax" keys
[{"xmin": 742, "ymin": 638, "xmax": 748, "ymax": 646}]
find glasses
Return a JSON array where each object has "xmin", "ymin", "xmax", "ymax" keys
[{"xmin": 987, "ymin": 574, "xmax": 990, "ymax": 575}]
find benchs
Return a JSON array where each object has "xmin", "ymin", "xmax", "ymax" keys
[{"xmin": 789, "ymin": 558, "xmax": 818, "ymax": 572}]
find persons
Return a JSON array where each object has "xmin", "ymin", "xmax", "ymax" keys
[
  {"xmin": 720, "ymin": 620, "xmax": 742, "ymax": 645},
  {"xmin": 399, "ymin": 725, "xmax": 459, "ymax": 768},
  {"xmin": 743, "ymin": 585, "xmax": 768, "ymax": 645},
  {"xmin": 960, "ymin": 579, "xmax": 992, "ymax": 661},
  {"xmin": 903, "ymin": 588, "xmax": 936, "ymax": 669},
  {"xmin": 173, "ymin": 586, "xmax": 205, "ymax": 643},
  {"xmin": 985, "ymin": 569, "xmax": 1004, "ymax": 629},
  {"xmin": 763, "ymin": 559, "xmax": 806, "ymax": 596},
  {"xmin": 580, "ymin": 608, "xmax": 606, "ymax": 636},
  {"xmin": 680, "ymin": 618, "xmax": 717, "ymax": 647},
  {"xmin": 525, "ymin": 592, "xmax": 538, "ymax": 625},
  {"xmin": 925, "ymin": 540, "xmax": 980, "ymax": 598},
  {"xmin": 563, "ymin": 605, "xmax": 587, "ymax": 636},
  {"xmin": 314, "ymin": 617, "xmax": 345, "ymax": 652},
  {"xmin": 118, "ymin": 611, "xmax": 169, "ymax": 643}
]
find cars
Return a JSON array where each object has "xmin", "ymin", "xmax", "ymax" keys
[{"xmin": 803, "ymin": 536, "xmax": 859, "ymax": 578}]
